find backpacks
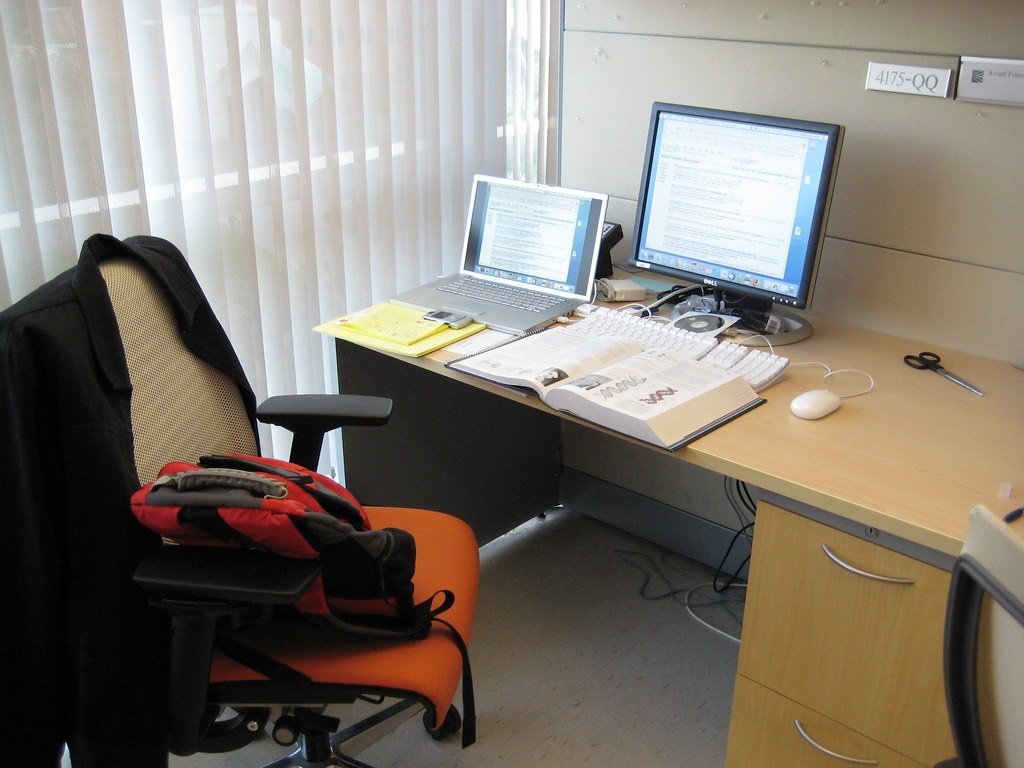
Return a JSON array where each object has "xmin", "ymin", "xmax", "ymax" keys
[{"xmin": 129, "ymin": 453, "xmax": 433, "ymax": 644}]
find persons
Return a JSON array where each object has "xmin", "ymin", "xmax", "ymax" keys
[{"xmin": 534, "ymin": 369, "xmax": 563, "ymax": 385}]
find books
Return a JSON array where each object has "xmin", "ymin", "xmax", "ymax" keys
[{"xmin": 331, "ymin": 303, "xmax": 768, "ymax": 451}]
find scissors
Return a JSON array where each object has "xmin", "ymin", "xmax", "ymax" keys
[{"xmin": 904, "ymin": 351, "xmax": 983, "ymax": 396}]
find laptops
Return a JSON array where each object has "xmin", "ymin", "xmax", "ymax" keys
[{"xmin": 389, "ymin": 174, "xmax": 609, "ymax": 334}]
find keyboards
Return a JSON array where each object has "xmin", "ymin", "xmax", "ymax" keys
[{"xmin": 568, "ymin": 306, "xmax": 790, "ymax": 392}]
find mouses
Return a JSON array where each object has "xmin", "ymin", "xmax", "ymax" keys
[{"xmin": 790, "ymin": 390, "xmax": 840, "ymax": 420}]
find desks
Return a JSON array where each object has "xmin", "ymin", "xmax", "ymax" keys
[{"xmin": 334, "ymin": 268, "xmax": 1024, "ymax": 768}]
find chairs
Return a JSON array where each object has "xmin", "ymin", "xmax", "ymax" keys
[
  {"xmin": 0, "ymin": 234, "xmax": 482, "ymax": 768},
  {"xmin": 935, "ymin": 505, "xmax": 1024, "ymax": 768}
]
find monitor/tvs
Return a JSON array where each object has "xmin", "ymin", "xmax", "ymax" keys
[{"xmin": 630, "ymin": 101, "xmax": 846, "ymax": 348}]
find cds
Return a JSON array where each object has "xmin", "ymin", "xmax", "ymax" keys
[{"xmin": 673, "ymin": 315, "xmax": 725, "ymax": 332}]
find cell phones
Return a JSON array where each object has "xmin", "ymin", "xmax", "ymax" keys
[{"xmin": 423, "ymin": 311, "xmax": 473, "ymax": 330}]
